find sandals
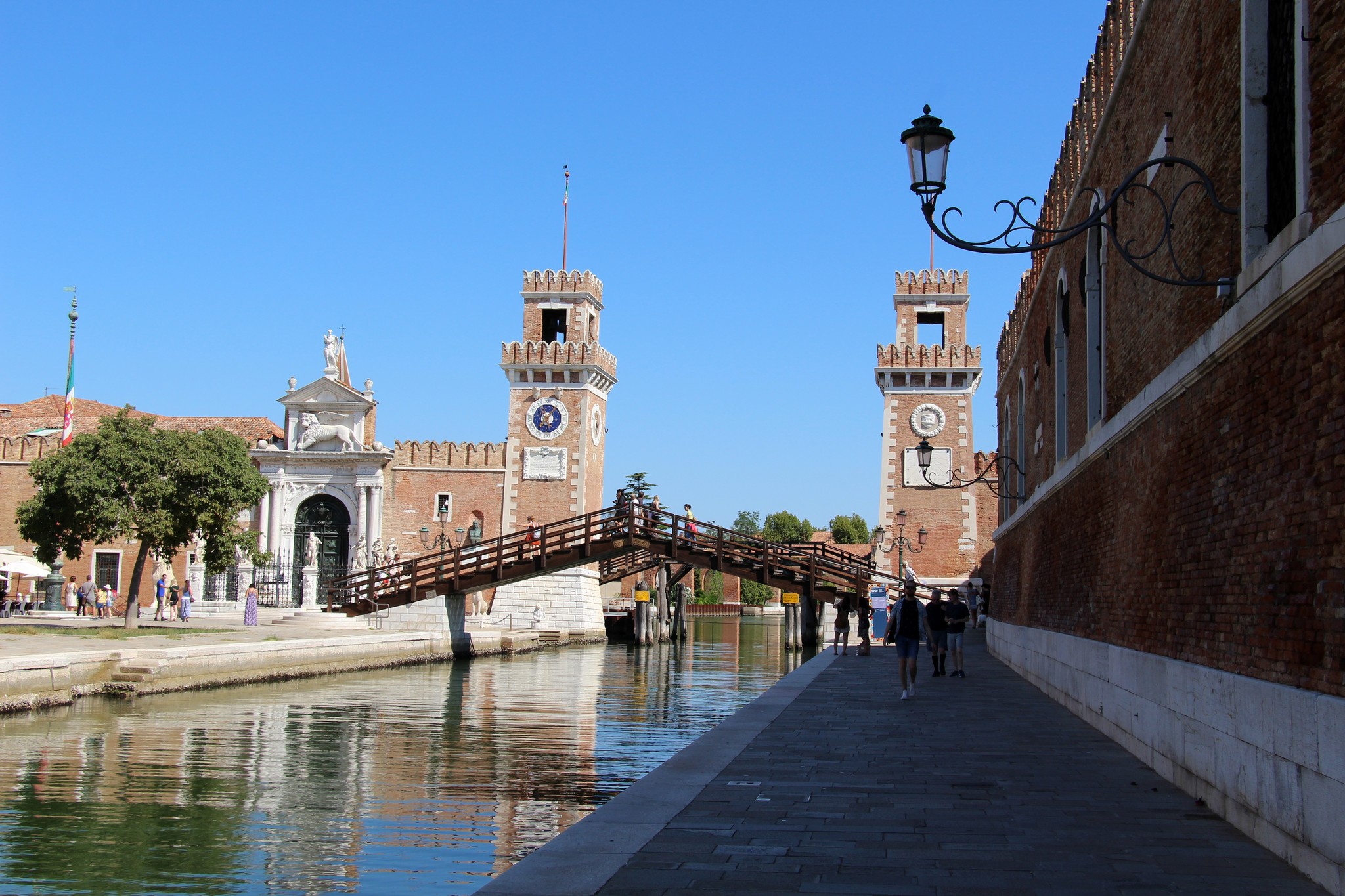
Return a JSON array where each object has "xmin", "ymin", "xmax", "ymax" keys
[
  {"xmin": 170, "ymin": 618, "xmax": 173, "ymax": 621},
  {"xmin": 857, "ymin": 652, "xmax": 870, "ymax": 656},
  {"xmin": 834, "ymin": 652, "xmax": 849, "ymax": 656},
  {"xmin": 174, "ymin": 619, "xmax": 177, "ymax": 621}
]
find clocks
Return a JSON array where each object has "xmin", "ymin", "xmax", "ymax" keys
[
  {"xmin": 525, "ymin": 397, "xmax": 569, "ymax": 440},
  {"xmin": 591, "ymin": 403, "xmax": 602, "ymax": 446}
]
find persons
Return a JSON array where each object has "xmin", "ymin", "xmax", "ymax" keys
[
  {"xmin": 324, "ymin": 329, "xmax": 339, "ymax": 370},
  {"xmin": 375, "ymin": 554, "xmax": 404, "ymax": 598},
  {"xmin": 514, "ymin": 515, "xmax": 545, "ymax": 559},
  {"xmin": 612, "ymin": 489, "xmax": 662, "ymax": 538},
  {"xmin": 154, "ymin": 574, "xmax": 193, "ymax": 622},
  {"xmin": 296, "ymin": 537, "xmax": 301, "ymax": 561},
  {"xmin": 470, "ymin": 520, "xmax": 482, "ymax": 538},
  {"xmin": 244, "ymin": 583, "xmax": 259, "ymax": 625},
  {"xmin": 284, "ymin": 482, "xmax": 303, "ymax": 510},
  {"xmin": 305, "ymin": 532, "xmax": 322, "ymax": 566},
  {"xmin": 66, "ymin": 575, "xmax": 113, "ymax": 621},
  {"xmin": 351, "ymin": 533, "xmax": 399, "ymax": 569},
  {"xmin": 682, "ymin": 504, "xmax": 698, "ymax": 548},
  {"xmin": 883, "ymin": 579, "xmax": 938, "ymax": 700},
  {"xmin": 832, "ymin": 561, "xmax": 991, "ymax": 678}
]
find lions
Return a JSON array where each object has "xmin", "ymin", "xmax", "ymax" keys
[
  {"xmin": 471, "ymin": 591, "xmax": 488, "ymax": 617},
  {"xmin": 296, "ymin": 412, "xmax": 372, "ymax": 451},
  {"xmin": 150, "ymin": 548, "xmax": 179, "ymax": 608}
]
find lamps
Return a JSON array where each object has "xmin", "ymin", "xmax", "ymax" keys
[
  {"xmin": 916, "ymin": 437, "xmax": 1026, "ymax": 500},
  {"xmin": 901, "ymin": 104, "xmax": 1239, "ymax": 290}
]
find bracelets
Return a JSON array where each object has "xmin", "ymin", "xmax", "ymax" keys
[{"xmin": 931, "ymin": 640, "xmax": 934, "ymax": 643}]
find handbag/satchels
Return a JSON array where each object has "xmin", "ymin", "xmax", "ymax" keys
[
  {"xmin": 887, "ymin": 618, "xmax": 897, "ymax": 644},
  {"xmin": 83, "ymin": 597, "xmax": 86, "ymax": 604},
  {"xmin": 976, "ymin": 589, "xmax": 985, "ymax": 605},
  {"xmin": 189, "ymin": 587, "xmax": 195, "ymax": 602},
  {"xmin": 857, "ymin": 642, "xmax": 871, "ymax": 656},
  {"xmin": 971, "ymin": 604, "xmax": 977, "ymax": 609}
]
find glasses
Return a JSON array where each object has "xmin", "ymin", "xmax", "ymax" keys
[
  {"xmin": 905, "ymin": 585, "xmax": 917, "ymax": 590},
  {"xmin": 163, "ymin": 577, "xmax": 166, "ymax": 579},
  {"xmin": 932, "ymin": 598, "xmax": 940, "ymax": 601}
]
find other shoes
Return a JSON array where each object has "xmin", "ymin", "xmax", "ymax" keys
[
  {"xmin": 958, "ymin": 670, "xmax": 965, "ymax": 678},
  {"xmin": 932, "ymin": 666, "xmax": 946, "ymax": 677},
  {"xmin": 615, "ymin": 530, "xmax": 621, "ymax": 534},
  {"xmin": 92, "ymin": 615, "xmax": 111, "ymax": 620},
  {"xmin": 683, "ymin": 543, "xmax": 698, "ymax": 548},
  {"xmin": 182, "ymin": 620, "xmax": 185, "ymax": 622},
  {"xmin": 949, "ymin": 671, "xmax": 959, "ymax": 677},
  {"xmin": 972, "ymin": 626, "xmax": 975, "ymax": 629},
  {"xmin": 154, "ymin": 617, "xmax": 158, "ymax": 621},
  {"xmin": 161, "ymin": 617, "xmax": 168, "ymax": 621},
  {"xmin": 185, "ymin": 617, "xmax": 188, "ymax": 622}
]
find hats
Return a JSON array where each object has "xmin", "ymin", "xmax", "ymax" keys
[{"xmin": 104, "ymin": 584, "xmax": 112, "ymax": 589}]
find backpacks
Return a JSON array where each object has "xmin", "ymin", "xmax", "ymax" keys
[
  {"xmin": 531, "ymin": 522, "xmax": 540, "ymax": 539},
  {"xmin": 624, "ymin": 500, "xmax": 634, "ymax": 514},
  {"xmin": 107, "ymin": 590, "xmax": 117, "ymax": 600},
  {"xmin": 835, "ymin": 604, "xmax": 849, "ymax": 629}
]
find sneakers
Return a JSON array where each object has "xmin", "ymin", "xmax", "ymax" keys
[{"xmin": 901, "ymin": 682, "xmax": 916, "ymax": 699}]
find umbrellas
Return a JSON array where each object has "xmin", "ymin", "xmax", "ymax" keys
[{"xmin": 0, "ymin": 549, "xmax": 67, "ymax": 610}]
